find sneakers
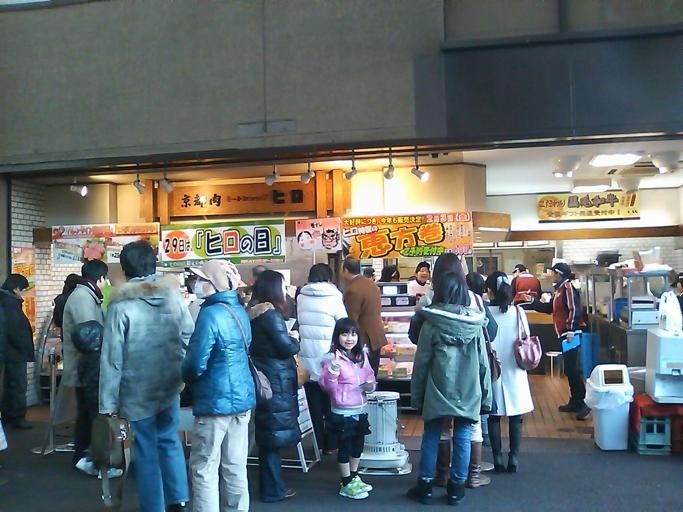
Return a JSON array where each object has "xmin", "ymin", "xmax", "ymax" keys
[
  {"xmin": 10, "ymin": 420, "xmax": 33, "ymax": 428},
  {"xmin": 75, "ymin": 458, "xmax": 123, "ymax": 478},
  {"xmin": 339, "ymin": 475, "xmax": 373, "ymax": 499}
]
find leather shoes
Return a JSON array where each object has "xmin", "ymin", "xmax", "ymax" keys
[
  {"xmin": 318, "ymin": 448, "xmax": 338, "ymax": 454},
  {"xmin": 261, "ymin": 487, "xmax": 295, "ymax": 501}
]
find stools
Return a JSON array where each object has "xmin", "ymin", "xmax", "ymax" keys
[{"xmin": 545, "ymin": 351, "xmax": 565, "ymax": 377}]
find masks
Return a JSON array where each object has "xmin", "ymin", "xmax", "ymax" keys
[{"xmin": 195, "ymin": 281, "xmax": 217, "ymax": 299}]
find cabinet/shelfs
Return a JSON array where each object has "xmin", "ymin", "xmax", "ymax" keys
[
  {"xmin": 37, "ymin": 313, "xmax": 64, "ymax": 402},
  {"xmin": 524, "ymin": 309, "xmax": 566, "ymax": 374},
  {"xmin": 377, "ymin": 306, "xmax": 426, "ymax": 414},
  {"xmin": 584, "ymin": 270, "xmax": 669, "ymax": 367}
]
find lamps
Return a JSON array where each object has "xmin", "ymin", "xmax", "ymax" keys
[
  {"xmin": 411, "ymin": 145, "xmax": 429, "ymax": 182},
  {"xmin": 264, "ymin": 164, "xmax": 281, "ymax": 186},
  {"xmin": 70, "ymin": 176, "xmax": 88, "ymax": 197},
  {"xmin": 160, "ymin": 173, "xmax": 173, "ymax": 193},
  {"xmin": 571, "ymin": 178, "xmax": 612, "ymax": 193},
  {"xmin": 384, "ymin": 145, "xmax": 394, "ymax": 179},
  {"xmin": 300, "ymin": 161, "xmax": 315, "ymax": 185},
  {"xmin": 345, "ymin": 147, "xmax": 358, "ymax": 179},
  {"xmin": 132, "ymin": 173, "xmax": 146, "ymax": 194}
]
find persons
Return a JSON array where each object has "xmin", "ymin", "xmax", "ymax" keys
[
  {"xmin": 522, "ymin": 263, "xmax": 592, "ymax": 420},
  {"xmin": 408, "ymin": 272, "xmax": 493, "ymax": 506},
  {"xmin": 98, "ymin": 241, "xmax": 195, "ymax": 512},
  {"xmin": 416, "ymin": 253, "xmax": 499, "ymax": 488},
  {"xmin": 0, "ymin": 274, "xmax": 36, "ymax": 429},
  {"xmin": 669, "ymin": 272, "xmax": 683, "ymax": 313},
  {"xmin": 318, "ymin": 318, "xmax": 377, "ymax": 501},
  {"xmin": 245, "ymin": 269, "xmax": 303, "ymax": 502},
  {"xmin": 53, "ymin": 241, "xmax": 542, "ymax": 479},
  {"xmin": 182, "ymin": 258, "xmax": 257, "ymax": 512}
]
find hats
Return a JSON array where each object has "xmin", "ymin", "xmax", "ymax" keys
[
  {"xmin": 190, "ymin": 260, "xmax": 238, "ymax": 292},
  {"xmin": 546, "ymin": 263, "xmax": 571, "ymax": 275},
  {"xmin": 671, "ymin": 273, "xmax": 683, "ymax": 287},
  {"xmin": 512, "ymin": 264, "xmax": 526, "ymax": 273}
]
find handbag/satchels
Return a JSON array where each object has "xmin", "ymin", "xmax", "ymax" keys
[
  {"xmin": 486, "ymin": 342, "xmax": 501, "ymax": 383},
  {"xmin": 515, "ymin": 335, "xmax": 542, "ymax": 370},
  {"xmin": 253, "ymin": 370, "xmax": 272, "ymax": 400},
  {"xmin": 90, "ymin": 416, "xmax": 136, "ymax": 470},
  {"xmin": 293, "ymin": 354, "xmax": 309, "ymax": 387}
]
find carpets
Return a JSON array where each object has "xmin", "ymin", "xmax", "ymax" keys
[{"xmin": 0, "ymin": 419, "xmax": 683, "ymax": 512}]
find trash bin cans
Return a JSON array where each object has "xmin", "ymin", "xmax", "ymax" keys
[{"xmin": 585, "ymin": 364, "xmax": 633, "ymax": 450}]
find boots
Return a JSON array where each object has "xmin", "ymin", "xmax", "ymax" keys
[
  {"xmin": 508, "ymin": 419, "xmax": 522, "ymax": 472},
  {"xmin": 559, "ymin": 397, "xmax": 591, "ymax": 419},
  {"xmin": 488, "ymin": 418, "xmax": 506, "ymax": 473},
  {"xmin": 408, "ymin": 434, "xmax": 490, "ymax": 505}
]
